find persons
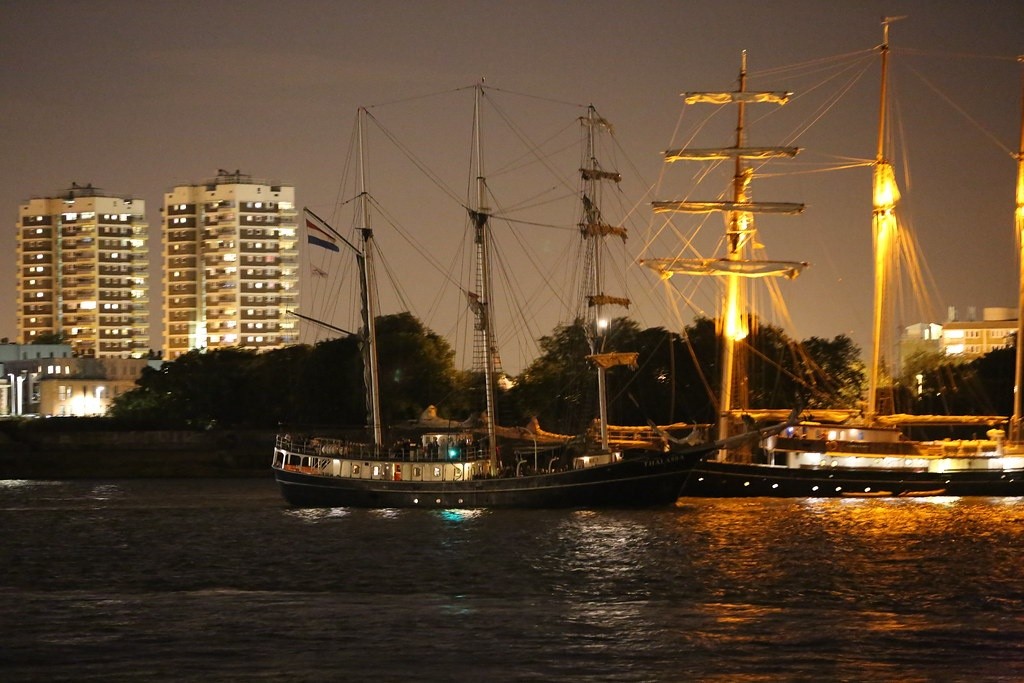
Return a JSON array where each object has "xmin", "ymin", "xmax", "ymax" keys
[
  {"xmin": 792, "ymin": 430, "xmax": 828, "ymax": 440},
  {"xmin": 284, "ymin": 432, "xmax": 323, "ymax": 457},
  {"xmin": 385, "ymin": 438, "xmax": 483, "ymax": 461},
  {"xmin": 473, "ymin": 465, "xmax": 570, "ymax": 480}
]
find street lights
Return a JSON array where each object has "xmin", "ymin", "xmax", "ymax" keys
[
  {"xmin": 97, "ymin": 386, "xmax": 106, "ymax": 414},
  {"xmin": 6, "ymin": 372, "xmax": 15, "ymax": 417}
]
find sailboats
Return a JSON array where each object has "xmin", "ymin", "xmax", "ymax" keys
[{"xmin": 267, "ymin": 16, "xmax": 1024, "ymax": 508}]
